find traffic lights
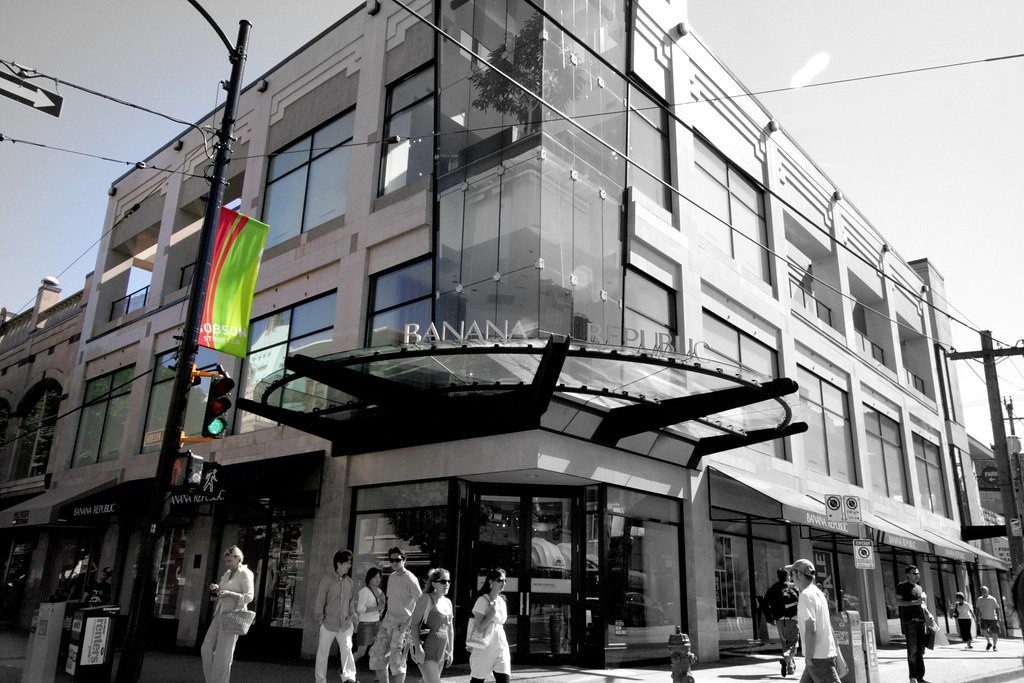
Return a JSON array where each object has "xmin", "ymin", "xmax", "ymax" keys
[
  {"xmin": 201, "ymin": 373, "xmax": 236, "ymax": 439},
  {"xmin": 196, "ymin": 460, "xmax": 227, "ymax": 497},
  {"xmin": 169, "ymin": 450, "xmax": 203, "ymax": 489}
]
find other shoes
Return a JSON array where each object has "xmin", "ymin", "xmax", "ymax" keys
[
  {"xmin": 918, "ymin": 679, "xmax": 931, "ymax": 683},
  {"xmin": 787, "ymin": 665, "xmax": 794, "ymax": 674},
  {"xmin": 986, "ymin": 643, "xmax": 992, "ymax": 650},
  {"xmin": 993, "ymin": 646, "xmax": 997, "ymax": 651},
  {"xmin": 779, "ymin": 658, "xmax": 788, "ymax": 677},
  {"xmin": 910, "ymin": 678, "xmax": 918, "ymax": 683}
]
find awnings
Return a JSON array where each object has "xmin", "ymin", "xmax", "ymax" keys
[
  {"xmin": 0, "ymin": 475, "xmax": 117, "ymax": 530},
  {"xmin": 58, "ymin": 450, "xmax": 325, "ymax": 527},
  {"xmin": 926, "ymin": 529, "xmax": 1012, "ymax": 573},
  {"xmin": 707, "ymin": 464, "xmax": 868, "ymax": 541},
  {"xmin": 879, "ymin": 514, "xmax": 977, "ymax": 565},
  {"xmin": 809, "ymin": 491, "xmax": 931, "ymax": 556}
]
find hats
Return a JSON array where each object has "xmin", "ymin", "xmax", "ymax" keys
[
  {"xmin": 785, "ymin": 558, "xmax": 815, "ymax": 578},
  {"xmin": 103, "ymin": 567, "xmax": 114, "ymax": 576}
]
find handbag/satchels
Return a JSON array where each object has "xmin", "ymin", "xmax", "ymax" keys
[
  {"xmin": 419, "ymin": 621, "xmax": 430, "ymax": 648},
  {"xmin": 835, "ymin": 644, "xmax": 849, "ymax": 678},
  {"xmin": 466, "ymin": 617, "xmax": 494, "ymax": 649},
  {"xmin": 925, "ymin": 620, "xmax": 949, "ymax": 650},
  {"xmin": 953, "ymin": 603, "xmax": 959, "ymax": 618},
  {"xmin": 221, "ymin": 594, "xmax": 256, "ymax": 635}
]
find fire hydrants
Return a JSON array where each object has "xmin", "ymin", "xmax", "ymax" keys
[{"xmin": 665, "ymin": 624, "xmax": 698, "ymax": 683}]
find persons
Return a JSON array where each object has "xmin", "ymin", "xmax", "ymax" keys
[
  {"xmin": 469, "ymin": 568, "xmax": 512, "ymax": 683},
  {"xmin": 895, "ymin": 565, "xmax": 934, "ymax": 683},
  {"xmin": 976, "ymin": 586, "xmax": 1000, "ymax": 651},
  {"xmin": 952, "ymin": 592, "xmax": 978, "ymax": 649},
  {"xmin": 353, "ymin": 567, "xmax": 385, "ymax": 683},
  {"xmin": 410, "ymin": 567, "xmax": 454, "ymax": 683},
  {"xmin": 71, "ymin": 553, "xmax": 98, "ymax": 600},
  {"xmin": 199, "ymin": 545, "xmax": 253, "ymax": 683},
  {"xmin": 761, "ymin": 558, "xmax": 843, "ymax": 683},
  {"xmin": 1011, "ymin": 567, "xmax": 1024, "ymax": 636},
  {"xmin": 313, "ymin": 549, "xmax": 359, "ymax": 683},
  {"xmin": 368, "ymin": 547, "xmax": 424, "ymax": 683},
  {"xmin": 80, "ymin": 567, "xmax": 113, "ymax": 608}
]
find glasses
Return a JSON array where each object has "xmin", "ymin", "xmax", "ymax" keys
[
  {"xmin": 912, "ymin": 572, "xmax": 920, "ymax": 575},
  {"xmin": 389, "ymin": 558, "xmax": 403, "ymax": 563},
  {"xmin": 493, "ymin": 578, "xmax": 507, "ymax": 583},
  {"xmin": 434, "ymin": 579, "xmax": 451, "ymax": 584}
]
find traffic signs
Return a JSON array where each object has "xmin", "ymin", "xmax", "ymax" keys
[{"xmin": 981, "ymin": 464, "xmax": 999, "ymax": 484}]
